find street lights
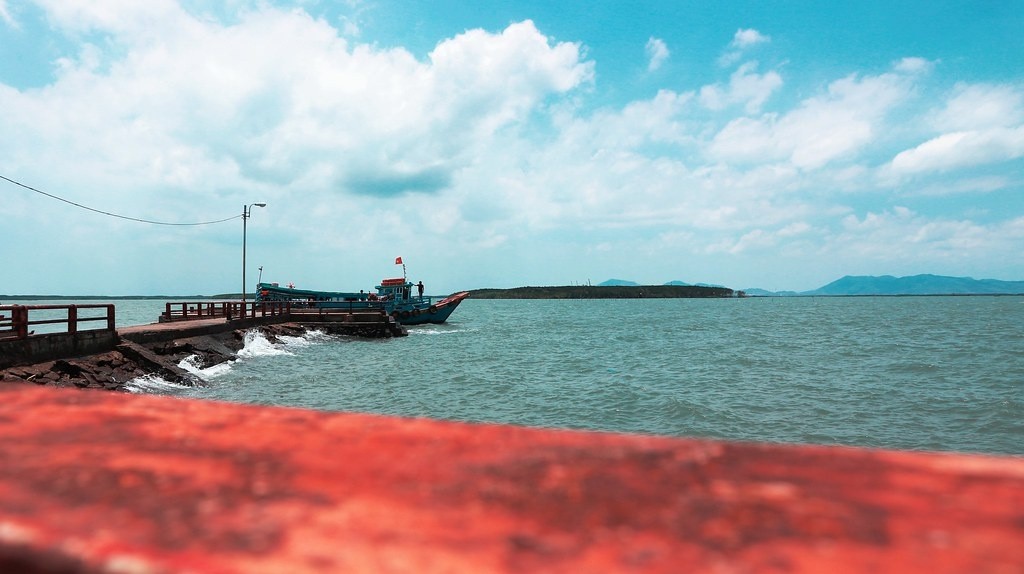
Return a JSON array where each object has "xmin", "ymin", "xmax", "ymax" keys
[{"xmin": 242, "ymin": 202, "xmax": 268, "ymax": 301}]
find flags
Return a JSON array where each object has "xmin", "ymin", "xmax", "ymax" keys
[{"xmin": 395, "ymin": 257, "xmax": 402, "ymax": 265}]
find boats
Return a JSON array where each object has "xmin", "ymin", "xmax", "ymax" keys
[{"xmin": 255, "ymin": 264, "xmax": 470, "ymax": 326}]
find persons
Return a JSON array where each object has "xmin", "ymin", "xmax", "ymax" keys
[{"xmin": 414, "ymin": 281, "xmax": 424, "ymax": 299}]
